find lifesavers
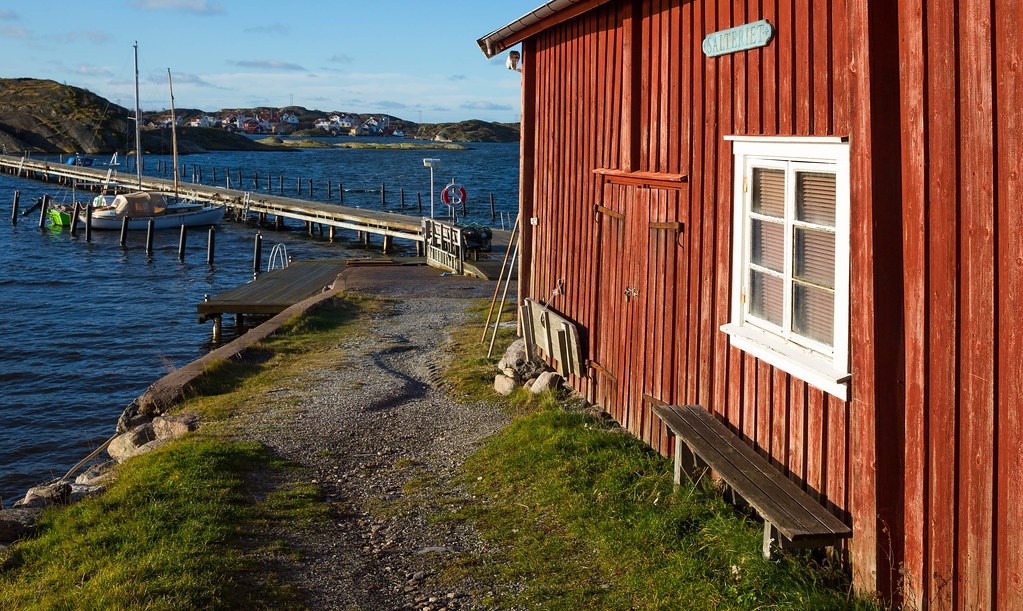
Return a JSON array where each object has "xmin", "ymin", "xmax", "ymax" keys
[{"xmin": 441, "ymin": 183, "xmax": 466, "ymax": 207}]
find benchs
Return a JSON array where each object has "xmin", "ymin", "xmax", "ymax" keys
[{"xmin": 650, "ymin": 404, "xmax": 854, "ymax": 562}]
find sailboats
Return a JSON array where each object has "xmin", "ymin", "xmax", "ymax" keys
[
  {"xmin": 80, "ymin": 66, "xmax": 227, "ymax": 231},
  {"xmin": 45, "ymin": 41, "xmax": 143, "ymax": 227}
]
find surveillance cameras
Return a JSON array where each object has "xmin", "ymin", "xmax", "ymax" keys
[{"xmin": 505, "ymin": 50, "xmax": 520, "ymax": 68}]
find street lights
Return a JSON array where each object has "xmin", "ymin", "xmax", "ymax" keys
[{"xmin": 422, "ymin": 157, "xmax": 442, "ymax": 219}]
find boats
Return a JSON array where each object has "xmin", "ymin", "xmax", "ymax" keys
[{"xmin": 63, "ymin": 150, "xmax": 100, "ymax": 168}]
